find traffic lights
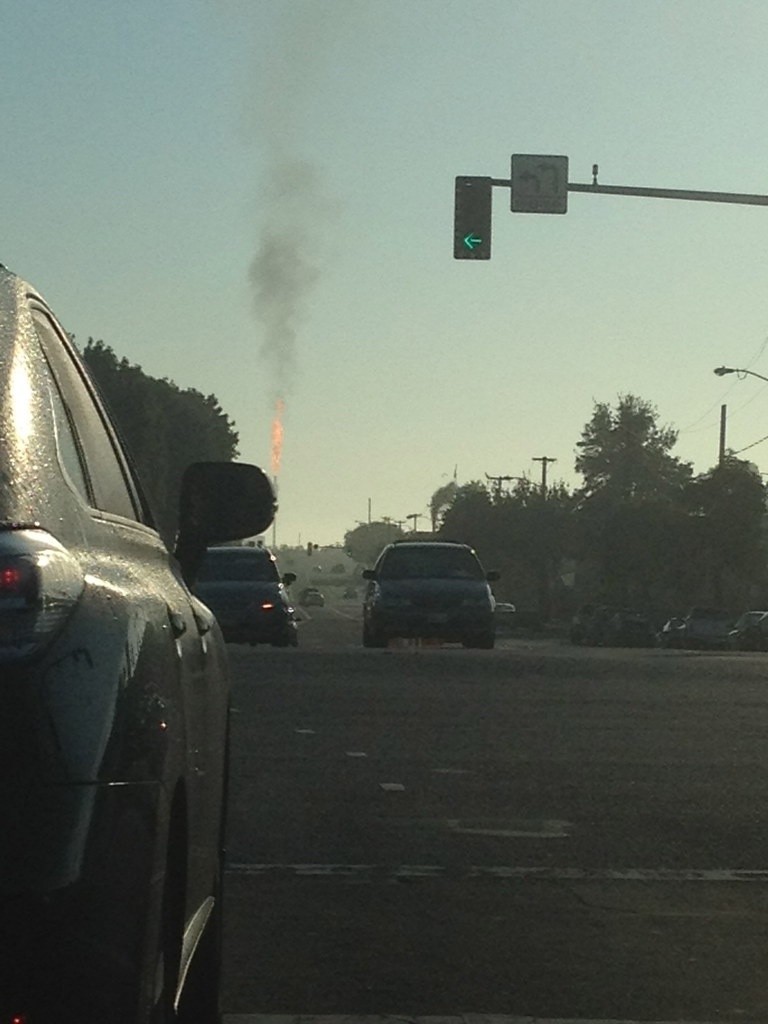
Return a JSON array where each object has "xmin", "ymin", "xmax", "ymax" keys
[
  {"xmin": 453, "ymin": 175, "xmax": 493, "ymax": 261},
  {"xmin": 308, "ymin": 542, "xmax": 313, "ymax": 556}
]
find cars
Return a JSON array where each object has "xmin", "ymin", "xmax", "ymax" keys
[
  {"xmin": 650, "ymin": 605, "xmax": 767, "ymax": 652},
  {"xmin": 362, "ymin": 539, "xmax": 503, "ymax": 651},
  {"xmin": 343, "ymin": 587, "xmax": 358, "ymax": 601},
  {"xmin": 287, "ymin": 605, "xmax": 301, "ymax": 645},
  {"xmin": 0, "ymin": 265, "xmax": 280, "ymax": 1024},
  {"xmin": 299, "ymin": 587, "xmax": 326, "ymax": 607}
]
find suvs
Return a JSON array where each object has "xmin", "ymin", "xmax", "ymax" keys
[{"xmin": 192, "ymin": 542, "xmax": 298, "ymax": 645}]
